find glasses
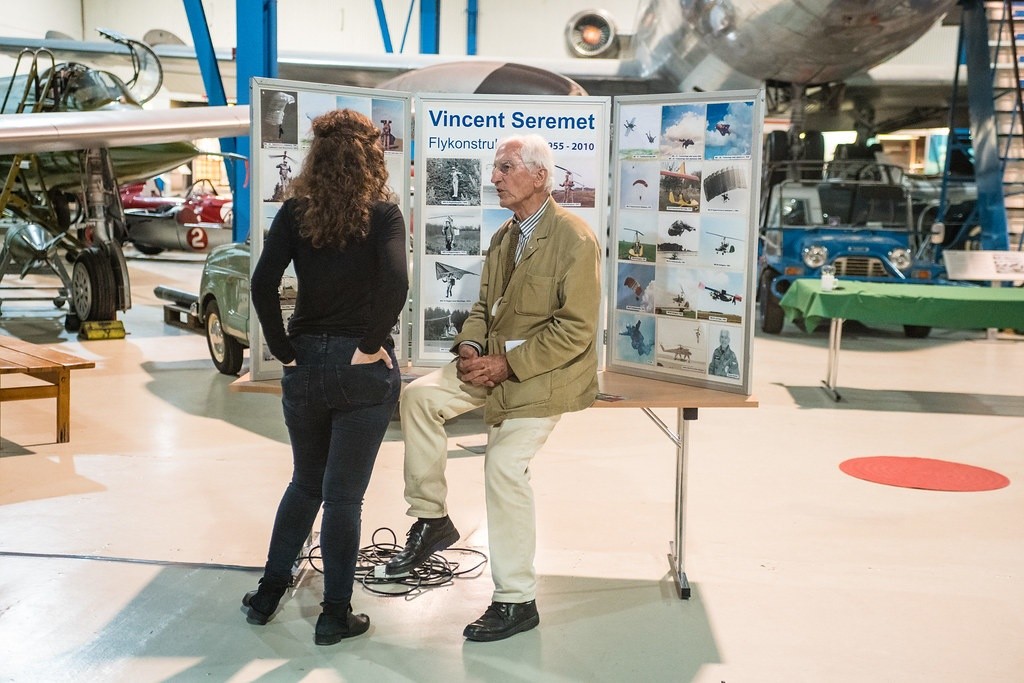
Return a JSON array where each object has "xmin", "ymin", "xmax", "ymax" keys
[{"xmin": 485, "ymin": 162, "xmax": 539, "ymax": 175}]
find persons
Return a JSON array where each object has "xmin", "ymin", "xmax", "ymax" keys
[
  {"xmin": 442, "ymin": 274, "xmax": 453, "ymax": 297},
  {"xmin": 276, "ymin": 161, "xmax": 291, "ymax": 193},
  {"xmin": 449, "ymin": 167, "xmax": 462, "ymax": 197},
  {"xmin": 242, "ymin": 110, "xmax": 409, "ymax": 647},
  {"xmin": 619, "ymin": 320, "xmax": 652, "ymax": 355},
  {"xmin": 442, "ymin": 221, "xmax": 455, "ymax": 251},
  {"xmin": 385, "ymin": 134, "xmax": 601, "ymax": 642},
  {"xmin": 709, "ymin": 329, "xmax": 739, "ymax": 378},
  {"xmin": 559, "ymin": 175, "xmax": 574, "ymax": 203}
]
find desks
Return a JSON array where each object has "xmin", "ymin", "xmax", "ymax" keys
[{"xmin": 228, "ymin": 361, "xmax": 759, "ymax": 600}]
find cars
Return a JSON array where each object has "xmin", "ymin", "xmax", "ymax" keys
[
  {"xmin": 114, "ymin": 168, "xmax": 237, "ymax": 254},
  {"xmin": 199, "ymin": 226, "xmax": 252, "ymax": 376}
]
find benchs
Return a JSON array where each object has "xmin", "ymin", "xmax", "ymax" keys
[
  {"xmin": 0, "ymin": 335, "xmax": 95, "ymax": 444},
  {"xmin": 817, "ymin": 184, "xmax": 908, "ymax": 227}
]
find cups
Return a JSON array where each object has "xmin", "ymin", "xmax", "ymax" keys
[{"xmin": 821, "ymin": 275, "xmax": 838, "ymax": 292}]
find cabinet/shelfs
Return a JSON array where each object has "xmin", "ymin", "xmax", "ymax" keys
[{"xmin": 778, "ymin": 278, "xmax": 1024, "ymax": 402}]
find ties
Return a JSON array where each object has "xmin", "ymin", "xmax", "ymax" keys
[{"xmin": 501, "ymin": 224, "xmax": 522, "ymax": 296}]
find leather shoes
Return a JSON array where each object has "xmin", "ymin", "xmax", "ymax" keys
[
  {"xmin": 385, "ymin": 514, "xmax": 460, "ymax": 574},
  {"xmin": 314, "ymin": 608, "xmax": 370, "ymax": 645},
  {"xmin": 462, "ymin": 599, "xmax": 539, "ymax": 641},
  {"xmin": 242, "ymin": 590, "xmax": 269, "ymax": 625}
]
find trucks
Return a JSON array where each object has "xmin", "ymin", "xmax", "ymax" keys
[{"xmin": 754, "ymin": 156, "xmax": 985, "ymax": 340}]
